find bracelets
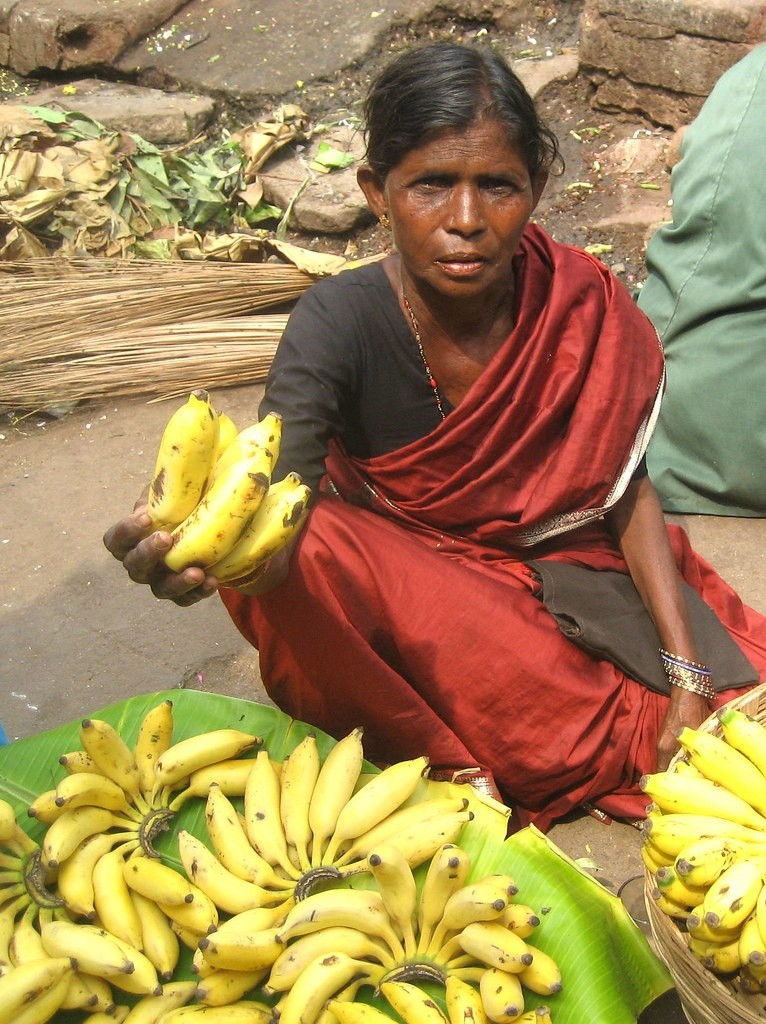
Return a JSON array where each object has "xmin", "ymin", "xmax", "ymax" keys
[{"xmin": 659, "ymin": 647, "xmax": 718, "ymax": 701}]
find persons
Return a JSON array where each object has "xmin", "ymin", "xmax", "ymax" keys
[
  {"xmin": 636, "ymin": 41, "xmax": 766, "ymax": 518},
  {"xmin": 100, "ymin": 38, "xmax": 766, "ymax": 843}
]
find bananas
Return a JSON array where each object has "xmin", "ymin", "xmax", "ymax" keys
[
  {"xmin": 136, "ymin": 388, "xmax": 310, "ymax": 592},
  {"xmin": 0, "ymin": 700, "xmax": 563, "ymax": 1023},
  {"xmin": 633, "ymin": 708, "xmax": 766, "ymax": 999}
]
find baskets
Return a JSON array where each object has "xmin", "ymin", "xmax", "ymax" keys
[{"xmin": 645, "ymin": 682, "xmax": 766, "ymax": 1024}]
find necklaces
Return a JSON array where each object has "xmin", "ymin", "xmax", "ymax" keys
[{"xmin": 401, "ymin": 288, "xmax": 446, "ymax": 420}]
